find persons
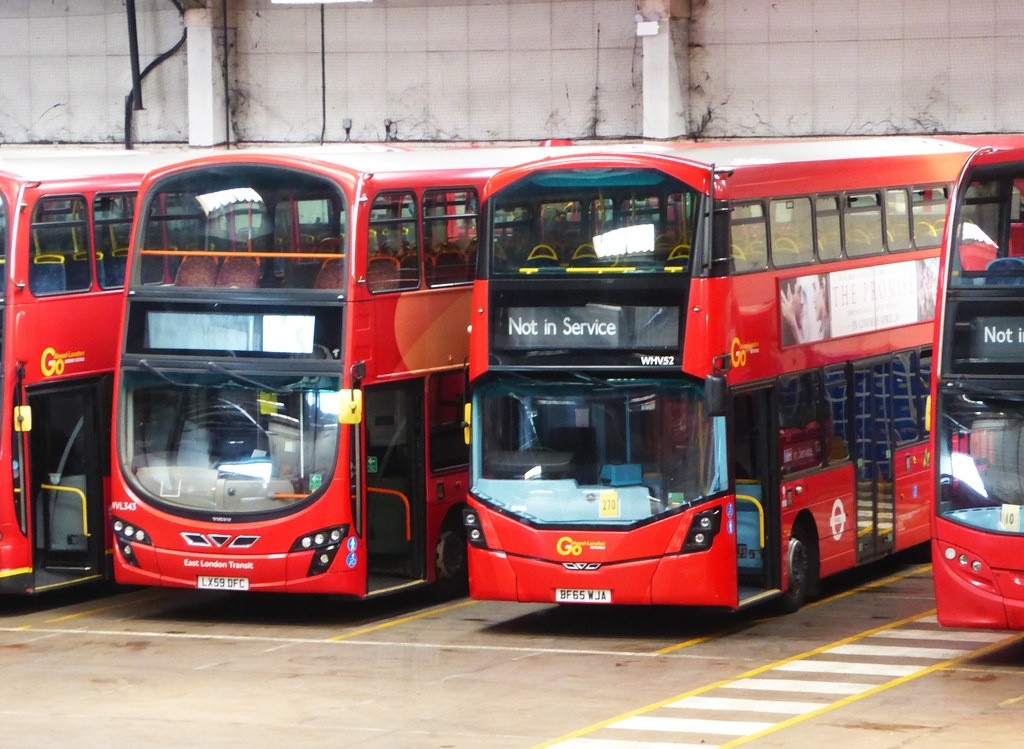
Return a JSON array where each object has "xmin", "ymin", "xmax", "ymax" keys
[{"xmin": 778, "ymin": 272, "xmax": 829, "ymax": 347}]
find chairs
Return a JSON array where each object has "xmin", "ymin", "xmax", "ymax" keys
[
  {"xmin": 984, "ymin": 258, "xmax": 1024, "ymax": 285},
  {"xmin": 545, "ymin": 427, "xmax": 597, "ymax": 484},
  {"xmin": 778, "ymin": 364, "xmax": 932, "ymax": 483},
  {"xmin": 0, "ymin": 219, "xmax": 959, "ymax": 296},
  {"xmin": 212, "ymin": 429, "xmax": 279, "ymax": 480}
]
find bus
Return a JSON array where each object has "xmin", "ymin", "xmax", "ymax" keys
[
  {"xmin": 1, "ymin": 141, "xmax": 682, "ymax": 607},
  {"xmin": 922, "ymin": 143, "xmax": 1024, "ymax": 632},
  {"xmin": 462, "ymin": 133, "xmax": 1024, "ymax": 624},
  {"xmin": 107, "ymin": 140, "xmax": 687, "ymax": 610}
]
